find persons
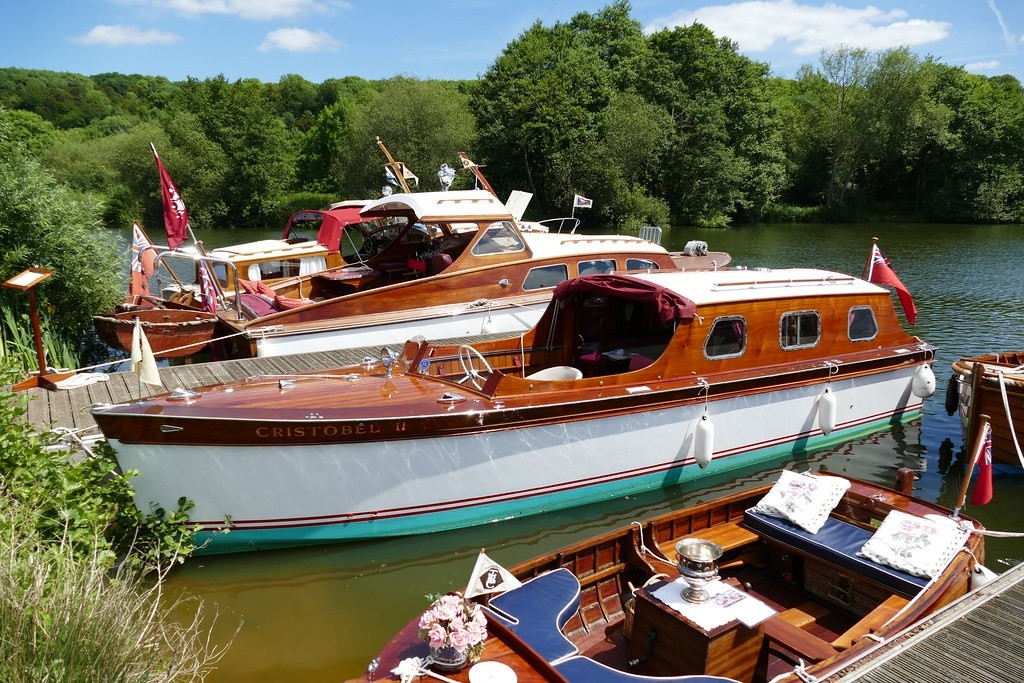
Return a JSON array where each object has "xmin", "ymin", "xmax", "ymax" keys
[{"xmin": 579, "ymin": 261, "xmax": 616, "ymax": 278}]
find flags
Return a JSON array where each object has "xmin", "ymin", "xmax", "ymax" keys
[
  {"xmin": 969, "ymin": 422, "xmax": 993, "ymax": 505},
  {"xmin": 867, "ymin": 243, "xmax": 918, "ymax": 327},
  {"xmin": 193, "ymin": 243, "xmax": 218, "ymax": 316},
  {"xmin": 157, "ymin": 157, "xmax": 188, "ymax": 249},
  {"xmin": 128, "ymin": 224, "xmax": 162, "ymax": 296},
  {"xmin": 574, "ymin": 195, "xmax": 593, "ymax": 208},
  {"xmin": 131, "ymin": 324, "xmax": 162, "ymax": 392}
]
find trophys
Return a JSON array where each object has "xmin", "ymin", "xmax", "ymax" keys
[{"xmin": 674, "ymin": 538, "xmax": 723, "ymax": 604}]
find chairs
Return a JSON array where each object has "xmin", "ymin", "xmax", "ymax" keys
[
  {"xmin": 430, "ymin": 252, "xmax": 451, "ymax": 274},
  {"xmin": 525, "ymin": 365, "xmax": 583, "ymax": 380}
]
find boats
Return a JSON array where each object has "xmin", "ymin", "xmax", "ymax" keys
[
  {"xmin": 951, "ymin": 348, "xmax": 1024, "ymax": 461},
  {"xmin": 343, "ymin": 468, "xmax": 988, "ymax": 683},
  {"xmin": 159, "ymin": 135, "xmax": 583, "ymax": 309},
  {"xmin": 205, "ymin": 198, "xmax": 407, "ymax": 265},
  {"xmin": 90, "ymin": 271, "xmax": 219, "ymax": 359},
  {"xmin": 215, "ymin": 153, "xmax": 734, "ymax": 359},
  {"xmin": 90, "ymin": 266, "xmax": 941, "ymax": 550}
]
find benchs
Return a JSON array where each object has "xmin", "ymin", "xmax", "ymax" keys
[
  {"xmin": 488, "ymin": 567, "xmax": 581, "ymax": 667},
  {"xmin": 743, "ymin": 507, "xmax": 929, "ymax": 598},
  {"xmin": 551, "ymin": 654, "xmax": 742, "ymax": 683},
  {"xmin": 831, "ymin": 592, "xmax": 911, "ymax": 647},
  {"xmin": 641, "ymin": 552, "xmax": 839, "ymax": 660},
  {"xmin": 659, "ymin": 516, "xmax": 759, "ymax": 563}
]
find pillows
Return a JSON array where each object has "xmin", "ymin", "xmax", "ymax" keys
[
  {"xmin": 855, "ymin": 510, "xmax": 971, "ymax": 580},
  {"xmin": 752, "ymin": 468, "xmax": 852, "ymax": 534}
]
